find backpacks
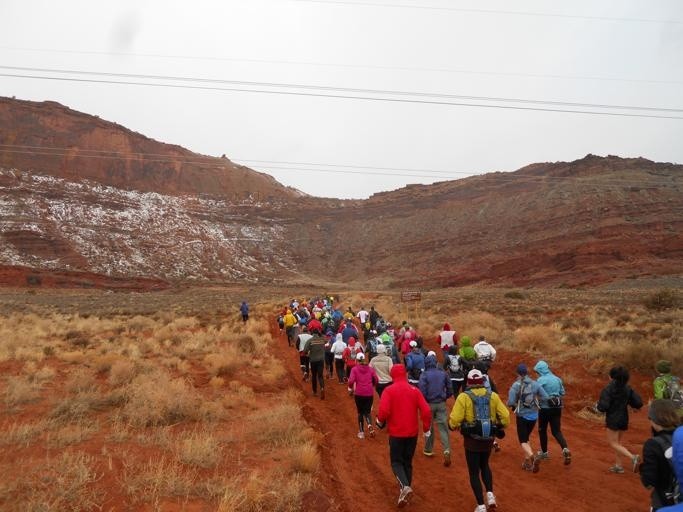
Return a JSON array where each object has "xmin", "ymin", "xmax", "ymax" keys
[
  {"xmin": 519, "ymin": 379, "xmax": 539, "ymax": 409},
  {"xmin": 658, "ymin": 376, "xmax": 683, "ymax": 408}
]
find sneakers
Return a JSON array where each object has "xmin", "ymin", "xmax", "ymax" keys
[
  {"xmin": 358, "ymin": 432, "xmax": 365, "ymax": 439},
  {"xmin": 474, "ymin": 505, "xmax": 487, "ymax": 512},
  {"xmin": 369, "ymin": 425, "xmax": 374, "ymax": 436},
  {"xmin": 444, "ymin": 451, "xmax": 451, "ymax": 466},
  {"xmin": 523, "ymin": 450, "xmax": 548, "ymax": 472},
  {"xmin": 494, "ymin": 442, "xmax": 501, "ymax": 452},
  {"xmin": 563, "ymin": 448, "xmax": 571, "ymax": 465},
  {"xmin": 609, "ymin": 465, "xmax": 624, "ymax": 473},
  {"xmin": 424, "ymin": 450, "xmax": 432, "ymax": 456},
  {"xmin": 632, "ymin": 455, "xmax": 640, "ymax": 473},
  {"xmin": 398, "ymin": 486, "xmax": 412, "ymax": 507},
  {"xmin": 487, "ymin": 492, "xmax": 496, "ymax": 507},
  {"xmin": 320, "ymin": 388, "xmax": 325, "ymax": 399}
]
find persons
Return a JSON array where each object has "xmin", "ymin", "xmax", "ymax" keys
[
  {"xmin": 655, "ymin": 426, "xmax": 682, "ymax": 512},
  {"xmin": 597, "ymin": 365, "xmax": 643, "ymax": 474},
  {"xmin": 653, "ymin": 360, "xmax": 681, "ymax": 400},
  {"xmin": 635, "ymin": 399, "xmax": 683, "ymax": 512},
  {"xmin": 240, "ymin": 301, "xmax": 249, "ymax": 322},
  {"xmin": 506, "ymin": 364, "xmax": 549, "ymax": 473},
  {"xmin": 533, "ymin": 361, "xmax": 571, "ymax": 465}
]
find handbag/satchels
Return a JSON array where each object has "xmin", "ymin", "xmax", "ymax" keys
[{"xmin": 548, "ymin": 398, "xmax": 561, "ymax": 407}]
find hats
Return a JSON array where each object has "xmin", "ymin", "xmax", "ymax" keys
[
  {"xmin": 410, "ymin": 340, "xmax": 417, "ymax": 347},
  {"xmin": 357, "ymin": 353, "xmax": 365, "ymax": 360},
  {"xmin": 377, "ymin": 344, "xmax": 385, "ymax": 354},
  {"xmin": 428, "ymin": 350, "xmax": 436, "ymax": 357},
  {"xmin": 467, "ymin": 369, "xmax": 483, "ymax": 379}
]
